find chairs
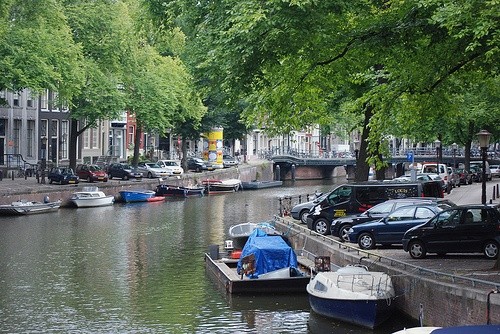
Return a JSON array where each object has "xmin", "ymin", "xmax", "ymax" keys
[{"xmin": 465, "ymin": 212, "xmax": 473, "ymax": 222}]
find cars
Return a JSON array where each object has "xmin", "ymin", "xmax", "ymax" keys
[
  {"xmin": 393, "ymin": 160, "xmax": 500, "ymax": 190},
  {"xmin": 345, "ymin": 203, "xmax": 464, "ymax": 251},
  {"xmin": 48, "ymin": 154, "xmax": 239, "ymax": 186},
  {"xmin": 291, "ymin": 189, "xmax": 351, "ymax": 225},
  {"xmin": 400, "ymin": 203, "xmax": 500, "ymax": 259}
]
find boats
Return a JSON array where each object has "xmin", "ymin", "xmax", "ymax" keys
[
  {"xmin": 393, "ymin": 323, "xmax": 500, "ymax": 334},
  {"xmin": 202, "ymin": 178, "xmax": 283, "ymax": 194},
  {"xmin": 118, "ymin": 189, "xmax": 156, "ymax": 202},
  {"xmin": 156, "ymin": 184, "xmax": 205, "ymax": 198},
  {"xmin": 0, "ymin": 196, "xmax": 63, "ymax": 216},
  {"xmin": 70, "ymin": 186, "xmax": 115, "ymax": 208},
  {"xmin": 229, "ymin": 221, "xmax": 288, "ymax": 250},
  {"xmin": 306, "ymin": 256, "xmax": 397, "ymax": 328}
]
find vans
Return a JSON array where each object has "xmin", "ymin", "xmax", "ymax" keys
[{"xmin": 306, "ymin": 181, "xmax": 446, "ymax": 236}]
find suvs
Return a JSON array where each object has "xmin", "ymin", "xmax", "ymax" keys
[{"xmin": 330, "ymin": 196, "xmax": 461, "ymax": 244}]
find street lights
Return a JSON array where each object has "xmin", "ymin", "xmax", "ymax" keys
[
  {"xmin": 475, "ymin": 129, "xmax": 493, "ymax": 221},
  {"xmin": 434, "ymin": 140, "xmax": 441, "ymax": 175},
  {"xmin": 41, "ymin": 136, "xmax": 48, "ymax": 184},
  {"xmin": 452, "ymin": 143, "xmax": 458, "ymax": 167},
  {"xmin": 478, "ymin": 145, "xmax": 482, "ymax": 159}
]
